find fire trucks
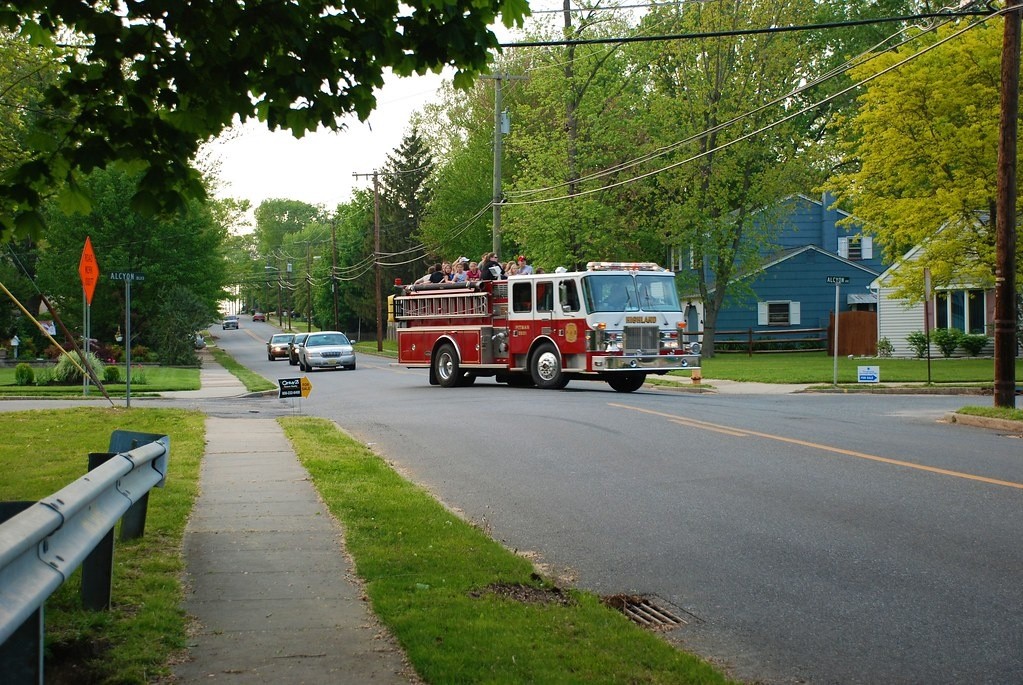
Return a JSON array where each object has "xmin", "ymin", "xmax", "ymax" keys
[{"xmin": 390, "ymin": 258, "xmax": 702, "ymax": 393}]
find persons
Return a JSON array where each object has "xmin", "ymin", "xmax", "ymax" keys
[
  {"xmin": 519, "ymin": 268, "xmax": 548, "ymax": 311},
  {"xmin": 414, "ymin": 252, "xmax": 532, "ymax": 285},
  {"xmin": 605, "ymin": 285, "xmax": 660, "ymax": 306}
]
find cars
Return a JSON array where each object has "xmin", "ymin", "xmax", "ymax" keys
[
  {"xmin": 266, "ymin": 333, "xmax": 297, "ymax": 361},
  {"xmin": 221, "ymin": 315, "xmax": 239, "ymax": 330},
  {"xmin": 288, "ymin": 332, "xmax": 327, "ymax": 365},
  {"xmin": 253, "ymin": 312, "xmax": 265, "ymax": 322},
  {"xmin": 266, "ymin": 310, "xmax": 300, "ymax": 318},
  {"xmin": 298, "ymin": 330, "xmax": 357, "ymax": 372}
]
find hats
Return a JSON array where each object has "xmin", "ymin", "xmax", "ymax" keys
[
  {"xmin": 459, "ymin": 257, "xmax": 469, "ymax": 263},
  {"xmin": 517, "ymin": 256, "xmax": 526, "ymax": 261}
]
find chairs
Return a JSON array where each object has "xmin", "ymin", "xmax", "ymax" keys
[
  {"xmin": 541, "ymin": 284, "xmax": 553, "ymax": 310},
  {"xmin": 276, "ymin": 340, "xmax": 281, "ymax": 342},
  {"xmin": 312, "ymin": 340, "xmax": 319, "ymax": 345}
]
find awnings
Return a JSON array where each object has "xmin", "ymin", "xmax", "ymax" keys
[{"xmin": 848, "ymin": 294, "xmax": 877, "ymax": 303}]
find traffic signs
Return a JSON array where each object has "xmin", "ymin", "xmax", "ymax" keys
[
  {"xmin": 857, "ymin": 365, "xmax": 880, "ymax": 382},
  {"xmin": 277, "ymin": 376, "xmax": 312, "ymax": 399}
]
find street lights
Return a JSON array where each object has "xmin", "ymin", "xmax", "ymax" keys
[{"xmin": 264, "ymin": 266, "xmax": 282, "ymax": 326}]
